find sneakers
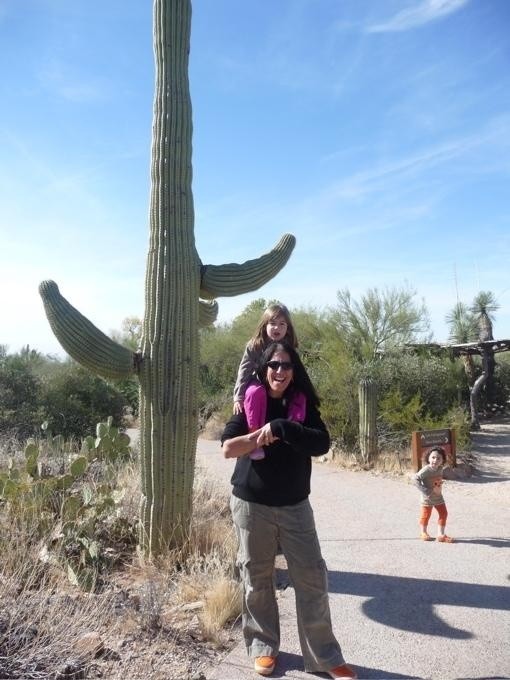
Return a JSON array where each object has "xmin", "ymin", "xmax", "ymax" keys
[
  {"xmin": 249, "ymin": 446, "xmax": 265, "ymax": 461},
  {"xmin": 327, "ymin": 663, "xmax": 357, "ymax": 679},
  {"xmin": 255, "ymin": 656, "xmax": 276, "ymax": 675}
]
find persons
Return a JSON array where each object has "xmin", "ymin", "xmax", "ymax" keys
[
  {"xmin": 220, "ymin": 343, "xmax": 361, "ymax": 680},
  {"xmin": 233, "ymin": 302, "xmax": 309, "ymax": 462},
  {"xmin": 411, "ymin": 445, "xmax": 457, "ymax": 544}
]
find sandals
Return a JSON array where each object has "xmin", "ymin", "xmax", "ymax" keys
[
  {"xmin": 437, "ymin": 535, "xmax": 455, "ymax": 543},
  {"xmin": 420, "ymin": 533, "xmax": 431, "ymax": 541}
]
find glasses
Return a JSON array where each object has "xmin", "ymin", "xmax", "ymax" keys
[{"xmin": 264, "ymin": 361, "xmax": 292, "ymax": 369}]
